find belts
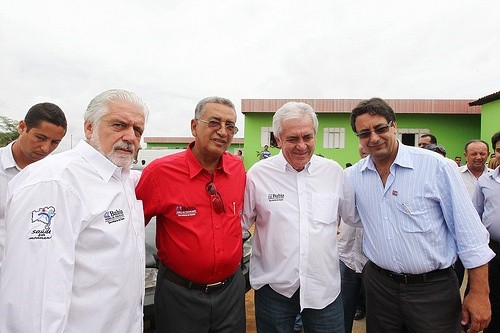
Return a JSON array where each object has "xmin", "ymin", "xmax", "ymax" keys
[
  {"xmin": 371, "ymin": 263, "xmax": 454, "ymax": 283},
  {"xmin": 158, "ymin": 263, "xmax": 235, "ymax": 294}
]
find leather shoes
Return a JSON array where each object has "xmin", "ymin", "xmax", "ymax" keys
[{"xmin": 353, "ymin": 310, "xmax": 365, "ymax": 320}]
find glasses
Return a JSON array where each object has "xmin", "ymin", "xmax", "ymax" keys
[
  {"xmin": 425, "ymin": 144, "xmax": 446, "ymax": 156},
  {"xmin": 206, "ymin": 181, "xmax": 225, "ymax": 215},
  {"xmin": 195, "ymin": 118, "xmax": 239, "ymax": 134},
  {"xmin": 355, "ymin": 120, "xmax": 392, "ymax": 139}
]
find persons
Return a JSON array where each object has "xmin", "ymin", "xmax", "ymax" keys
[
  {"xmin": 260, "ymin": 145, "xmax": 271, "ymax": 160},
  {"xmin": 345, "ymin": 97, "xmax": 496, "ymax": 333},
  {"xmin": 418, "ymin": 132, "xmax": 500, "ymax": 333},
  {"xmin": 0, "ymin": 102, "xmax": 67, "ymax": 270},
  {"xmin": 135, "ymin": 97, "xmax": 247, "ymax": 333},
  {"xmin": 294, "ymin": 143, "xmax": 370, "ymax": 333},
  {"xmin": 0, "ymin": 89, "xmax": 149, "ymax": 333},
  {"xmin": 241, "ymin": 102, "xmax": 363, "ymax": 333},
  {"xmin": 236, "ymin": 149, "xmax": 244, "ymax": 163}
]
